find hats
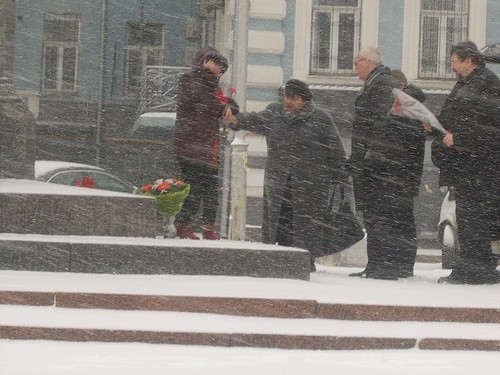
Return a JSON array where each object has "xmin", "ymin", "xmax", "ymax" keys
[
  {"xmin": 193, "ymin": 48, "xmax": 229, "ymax": 76},
  {"xmin": 285, "ymin": 79, "xmax": 313, "ymax": 100}
]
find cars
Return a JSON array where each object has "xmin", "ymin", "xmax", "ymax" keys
[
  {"xmin": 437, "ymin": 186, "xmax": 500, "ymax": 270},
  {"xmin": 35, "ymin": 159, "xmax": 140, "ymax": 194}
]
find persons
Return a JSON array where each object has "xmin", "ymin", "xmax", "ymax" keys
[
  {"xmin": 228, "ymin": 79, "xmax": 348, "ymax": 273},
  {"xmin": 390, "ymin": 69, "xmax": 427, "ymax": 278},
  {"xmin": 346, "ymin": 46, "xmax": 401, "ymax": 281},
  {"xmin": 173, "ymin": 47, "xmax": 240, "ymax": 241},
  {"xmin": 0, "ymin": 83, "xmax": 37, "ymax": 232},
  {"xmin": 423, "ymin": 40, "xmax": 500, "ymax": 285}
]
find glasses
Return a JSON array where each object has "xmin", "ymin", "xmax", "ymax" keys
[{"xmin": 354, "ymin": 58, "xmax": 365, "ymax": 66}]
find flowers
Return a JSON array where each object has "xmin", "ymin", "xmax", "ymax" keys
[
  {"xmin": 386, "ymin": 88, "xmax": 449, "ymax": 137},
  {"xmin": 139, "ymin": 178, "xmax": 191, "ymax": 238},
  {"xmin": 217, "ymin": 87, "xmax": 238, "ymax": 143},
  {"xmin": 76, "ymin": 176, "xmax": 95, "ymax": 188}
]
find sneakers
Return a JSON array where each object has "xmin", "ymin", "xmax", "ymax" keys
[
  {"xmin": 465, "ymin": 270, "xmax": 499, "ymax": 284},
  {"xmin": 349, "ymin": 263, "xmax": 374, "ymax": 277},
  {"xmin": 310, "ymin": 260, "xmax": 317, "ymax": 273},
  {"xmin": 364, "ymin": 263, "xmax": 400, "ymax": 280},
  {"xmin": 438, "ymin": 270, "xmax": 467, "ymax": 284},
  {"xmin": 176, "ymin": 221, "xmax": 200, "ymax": 239},
  {"xmin": 203, "ymin": 225, "xmax": 221, "ymax": 240}
]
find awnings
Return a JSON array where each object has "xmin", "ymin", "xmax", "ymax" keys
[{"xmin": 128, "ymin": 111, "xmax": 177, "ymax": 142}]
